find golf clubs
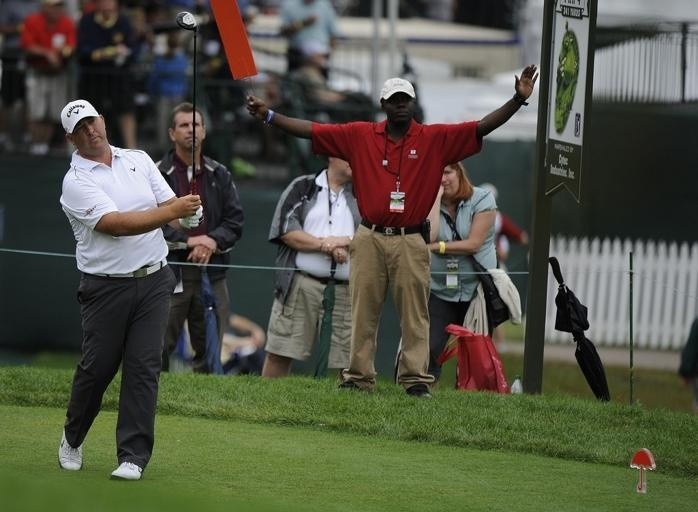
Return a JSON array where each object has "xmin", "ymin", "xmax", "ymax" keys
[{"xmin": 176, "ymin": 11, "xmax": 198, "ymax": 195}]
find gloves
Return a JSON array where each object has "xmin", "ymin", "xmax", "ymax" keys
[{"xmin": 178, "ymin": 204, "xmax": 205, "ymax": 229}]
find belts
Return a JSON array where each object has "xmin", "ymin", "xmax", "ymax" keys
[
  {"xmin": 95, "ymin": 257, "xmax": 168, "ymax": 277},
  {"xmin": 295, "ymin": 267, "xmax": 348, "ymax": 286},
  {"xmin": 360, "ymin": 218, "xmax": 421, "ymax": 235}
]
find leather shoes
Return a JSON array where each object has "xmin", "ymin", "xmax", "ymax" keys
[
  {"xmin": 338, "ymin": 381, "xmax": 358, "ymax": 389},
  {"xmin": 408, "ymin": 385, "xmax": 431, "ymax": 399}
]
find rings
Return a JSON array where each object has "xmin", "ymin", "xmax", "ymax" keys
[
  {"xmin": 201, "ymin": 253, "xmax": 206, "ymax": 257},
  {"xmin": 326, "ymin": 244, "xmax": 331, "ymax": 247}
]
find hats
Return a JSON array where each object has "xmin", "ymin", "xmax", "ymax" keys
[
  {"xmin": 380, "ymin": 78, "xmax": 416, "ymax": 103},
  {"xmin": 61, "ymin": 100, "xmax": 98, "ymax": 135}
]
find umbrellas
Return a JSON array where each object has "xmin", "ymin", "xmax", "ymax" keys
[{"xmin": 548, "ymin": 254, "xmax": 610, "ymax": 406}]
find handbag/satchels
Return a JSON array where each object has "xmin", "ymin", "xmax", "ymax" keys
[
  {"xmin": 477, "ymin": 269, "xmax": 511, "ymax": 329},
  {"xmin": 436, "ymin": 325, "xmax": 511, "ymax": 394}
]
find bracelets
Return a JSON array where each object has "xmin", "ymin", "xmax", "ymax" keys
[
  {"xmin": 348, "ymin": 236, "xmax": 353, "ymax": 241},
  {"xmin": 443, "ymin": 240, "xmax": 447, "ymax": 255},
  {"xmin": 438, "ymin": 240, "xmax": 444, "ymax": 255}
]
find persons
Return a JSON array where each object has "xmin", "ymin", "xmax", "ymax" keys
[
  {"xmin": 242, "ymin": 62, "xmax": 539, "ymax": 399},
  {"xmin": 58, "ymin": 97, "xmax": 203, "ymax": 478},
  {"xmin": 155, "ymin": 104, "xmax": 245, "ymax": 373},
  {"xmin": 260, "ymin": 157, "xmax": 363, "ymax": 377},
  {"xmin": 427, "ymin": 159, "xmax": 500, "ymax": 382},
  {"xmin": 1, "ymin": 0, "xmax": 375, "ymax": 175},
  {"xmin": 182, "ymin": 301, "xmax": 267, "ymax": 373}
]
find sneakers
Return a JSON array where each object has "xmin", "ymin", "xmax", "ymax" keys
[
  {"xmin": 58, "ymin": 428, "xmax": 82, "ymax": 471},
  {"xmin": 111, "ymin": 461, "xmax": 142, "ymax": 481}
]
